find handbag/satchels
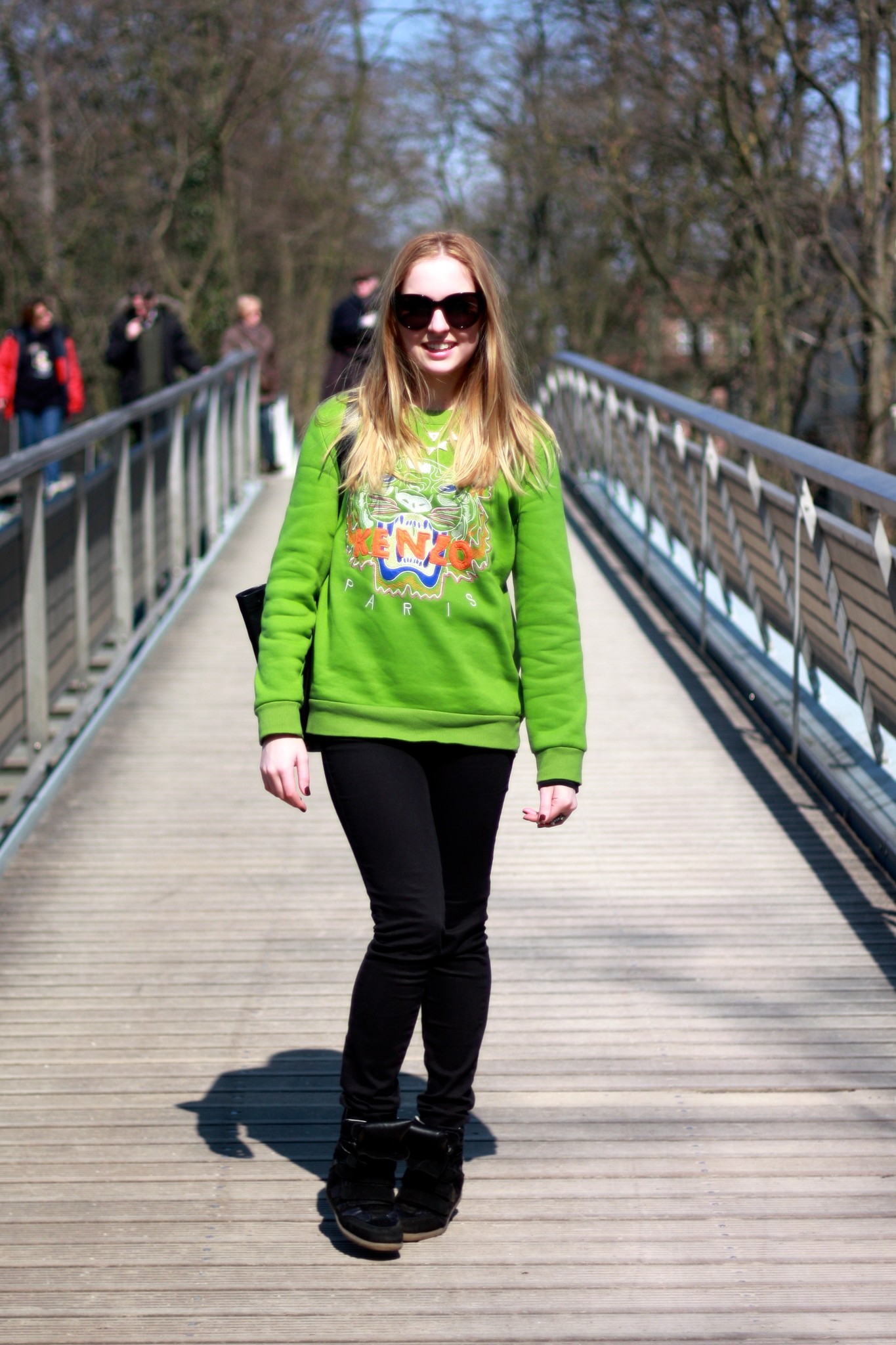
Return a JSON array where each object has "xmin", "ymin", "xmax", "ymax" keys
[{"xmin": 233, "ymin": 388, "xmax": 358, "ymax": 752}]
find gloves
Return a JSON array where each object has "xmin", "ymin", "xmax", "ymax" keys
[{"xmin": 358, "ymin": 312, "xmax": 379, "ymax": 330}]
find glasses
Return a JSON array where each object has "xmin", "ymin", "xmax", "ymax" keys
[{"xmin": 390, "ymin": 290, "xmax": 488, "ymax": 331}]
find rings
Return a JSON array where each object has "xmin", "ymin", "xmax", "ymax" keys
[{"xmin": 550, "ymin": 816, "xmax": 564, "ymax": 826}]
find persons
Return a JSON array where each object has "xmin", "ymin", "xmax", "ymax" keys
[
  {"xmin": 1, "ymin": 268, "xmax": 382, "ymax": 508},
  {"xmin": 253, "ymin": 230, "xmax": 588, "ymax": 1255}
]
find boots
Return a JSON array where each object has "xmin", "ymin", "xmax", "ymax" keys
[
  {"xmin": 325, "ymin": 1114, "xmax": 411, "ymax": 1253},
  {"xmin": 393, "ymin": 1115, "xmax": 465, "ymax": 1242}
]
